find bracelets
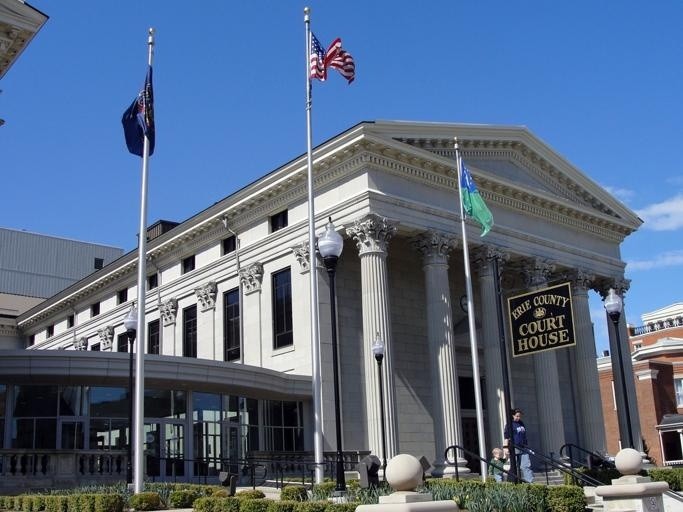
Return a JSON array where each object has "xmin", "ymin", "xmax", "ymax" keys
[{"xmin": 504, "ymin": 446, "xmax": 509, "ymax": 447}]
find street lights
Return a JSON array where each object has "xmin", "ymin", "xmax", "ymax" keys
[
  {"xmin": 315, "ymin": 217, "xmax": 352, "ymax": 496},
  {"xmin": 372, "ymin": 330, "xmax": 389, "ymax": 482},
  {"xmin": 602, "ymin": 285, "xmax": 637, "ymax": 450},
  {"xmin": 121, "ymin": 300, "xmax": 136, "ymax": 487}
]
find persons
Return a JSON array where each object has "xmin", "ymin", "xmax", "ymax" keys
[
  {"xmin": 504, "ymin": 408, "xmax": 535, "ymax": 483},
  {"xmin": 489, "ymin": 448, "xmax": 509, "ymax": 482}
]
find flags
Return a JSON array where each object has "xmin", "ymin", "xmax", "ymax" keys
[
  {"xmin": 307, "ymin": 30, "xmax": 356, "ymax": 103},
  {"xmin": 461, "ymin": 157, "xmax": 495, "ymax": 239},
  {"xmin": 121, "ymin": 65, "xmax": 155, "ymax": 158}
]
